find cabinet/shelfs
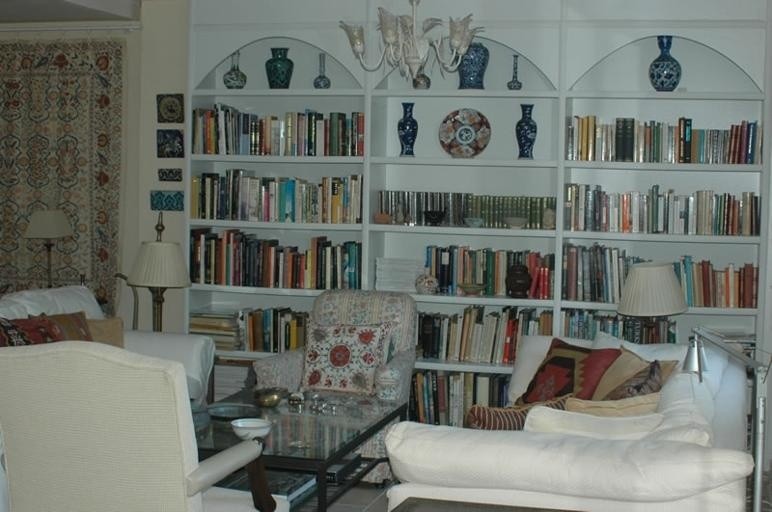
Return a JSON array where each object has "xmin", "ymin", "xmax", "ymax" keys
[{"xmin": 182, "ymin": 23, "xmax": 765, "ymax": 381}]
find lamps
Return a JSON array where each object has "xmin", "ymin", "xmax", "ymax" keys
[
  {"xmin": 128, "ymin": 207, "xmax": 191, "ymax": 333},
  {"xmin": 22, "ymin": 208, "xmax": 71, "ymax": 291},
  {"xmin": 618, "ymin": 262, "xmax": 688, "ymax": 343},
  {"xmin": 339, "ymin": 2, "xmax": 484, "ymax": 81}
]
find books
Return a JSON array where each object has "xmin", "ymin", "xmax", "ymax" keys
[
  {"xmin": 189, "ymin": 229, "xmax": 283, "ymax": 289},
  {"xmin": 562, "ymin": 243, "xmax": 653, "ymax": 304},
  {"xmin": 690, "ymin": 120, "xmax": 757, "ymax": 164},
  {"xmin": 670, "ymin": 255, "xmax": 759, "ymax": 309},
  {"xmin": 265, "ymin": 108, "xmax": 361, "ymax": 157},
  {"xmin": 614, "ymin": 117, "xmax": 692, "ymax": 163},
  {"xmin": 377, "ymin": 190, "xmax": 472, "ymax": 227},
  {"xmin": 562, "ymin": 308, "xmax": 677, "ymax": 345},
  {"xmin": 213, "ymin": 467, "xmax": 319, "ymax": 503},
  {"xmin": 491, "ymin": 305, "xmax": 552, "ymax": 365},
  {"xmin": 191, "ymin": 103, "xmax": 265, "ymax": 157},
  {"xmin": 261, "ymin": 174, "xmax": 361, "ymax": 225},
  {"xmin": 409, "ymin": 368, "xmax": 512, "ymax": 426},
  {"xmin": 564, "ymin": 183, "xmax": 653, "ymax": 234},
  {"xmin": 188, "ymin": 304, "xmax": 308, "ymax": 354},
  {"xmin": 567, "ymin": 115, "xmax": 617, "ymax": 162},
  {"xmin": 652, "ymin": 185, "xmax": 761, "ymax": 237},
  {"xmin": 412, "ymin": 305, "xmax": 502, "ymax": 365},
  {"xmin": 189, "ymin": 168, "xmax": 262, "ymax": 222},
  {"xmin": 689, "ymin": 335, "xmax": 756, "ymax": 358},
  {"xmin": 284, "ymin": 236, "xmax": 361, "ymax": 290},
  {"xmin": 260, "ymin": 412, "xmax": 366, "ymax": 467},
  {"xmin": 471, "ymin": 196, "xmax": 554, "ymax": 230},
  {"xmin": 425, "ymin": 245, "xmax": 553, "ymax": 300}
]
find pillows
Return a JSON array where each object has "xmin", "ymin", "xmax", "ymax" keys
[
  {"xmin": 299, "ymin": 316, "xmax": 392, "ymax": 395},
  {"xmin": 0, "ymin": 312, "xmax": 124, "ymax": 351},
  {"xmin": 464, "ymin": 338, "xmax": 678, "ymax": 430}
]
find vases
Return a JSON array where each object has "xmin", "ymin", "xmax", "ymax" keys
[
  {"xmin": 398, "ymin": 103, "xmax": 418, "ymax": 157},
  {"xmin": 224, "ymin": 50, "xmax": 247, "ymax": 89},
  {"xmin": 265, "ymin": 47, "xmax": 294, "ymax": 89},
  {"xmin": 515, "ymin": 104, "xmax": 538, "ymax": 160},
  {"xmin": 507, "ymin": 55, "xmax": 522, "ymax": 90},
  {"xmin": 413, "ymin": 65, "xmax": 431, "ymax": 89},
  {"xmin": 649, "ymin": 38, "xmax": 682, "ymax": 92},
  {"xmin": 452, "ymin": 40, "xmax": 490, "ymax": 90},
  {"xmin": 313, "ymin": 53, "xmax": 331, "ymax": 89}
]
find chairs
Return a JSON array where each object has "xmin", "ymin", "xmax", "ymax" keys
[
  {"xmin": 1, "ymin": 340, "xmax": 291, "ymax": 511},
  {"xmin": 245, "ymin": 286, "xmax": 418, "ymax": 485},
  {"xmin": 0, "ymin": 285, "xmax": 218, "ymax": 444}
]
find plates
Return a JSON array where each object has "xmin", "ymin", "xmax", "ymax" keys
[
  {"xmin": 457, "ymin": 283, "xmax": 489, "ymax": 295},
  {"xmin": 438, "ymin": 108, "xmax": 492, "ymax": 160}
]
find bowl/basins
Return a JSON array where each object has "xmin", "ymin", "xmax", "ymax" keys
[
  {"xmin": 203, "ymin": 403, "xmax": 262, "ymax": 433},
  {"xmin": 231, "ymin": 418, "xmax": 272, "ymax": 440},
  {"xmin": 254, "ymin": 388, "xmax": 281, "ymax": 407},
  {"xmin": 504, "ymin": 217, "xmax": 527, "ymax": 229},
  {"xmin": 464, "ymin": 218, "xmax": 482, "ymax": 227}
]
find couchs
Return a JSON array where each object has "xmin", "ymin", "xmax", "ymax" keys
[{"xmin": 385, "ymin": 322, "xmax": 753, "ymax": 510}]
